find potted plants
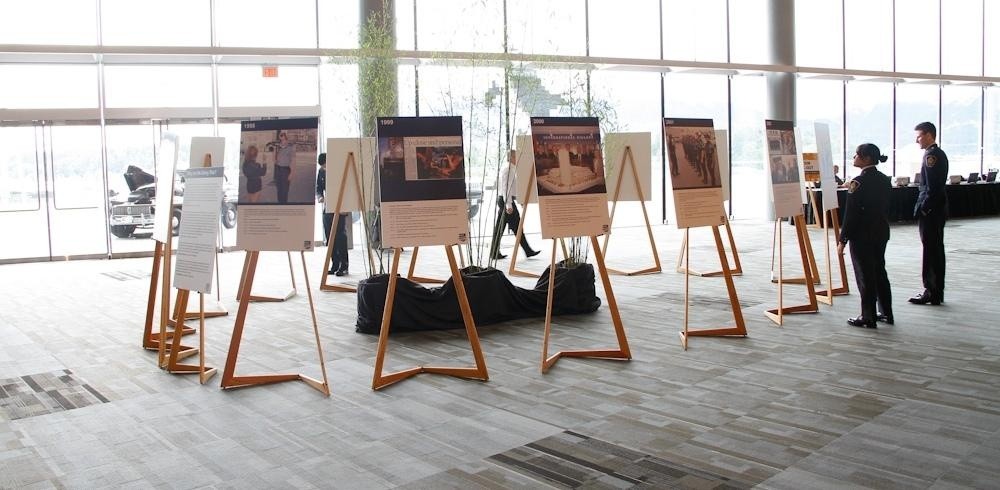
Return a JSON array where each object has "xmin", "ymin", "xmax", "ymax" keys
[
  {"xmin": 432, "ymin": 43, "xmax": 537, "ymax": 325},
  {"xmin": 338, "ymin": 1, "xmax": 401, "ymax": 332},
  {"xmin": 540, "ymin": 52, "xmax": 616, "ymax": 315}
]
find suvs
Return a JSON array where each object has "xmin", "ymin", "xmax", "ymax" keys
[{"xmin": 351, "ymin": 176, "xmax": 485, "ymax": 227}]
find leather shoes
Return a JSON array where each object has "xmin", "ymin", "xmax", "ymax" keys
[
  {"xmin": 917, "ymin": 292, "xmax": 944, "ymax": 303},
  {"xmin": 494, "ymin": 254, "xmax": 507, "ymax": 259},
  {"xmin": 848, "ymin": 316, "xmax": 877, "ymax": 328},
  {"xmin": 908, "ymin": 296, "xmax": 940, "ymax": 305},
  {"xmin": 527, "ymin": 251, "xmax": 540, "ymax": 257},
  {"xmin": 877, "ymin": 312, "xmax": 893, "ymax": 324}
]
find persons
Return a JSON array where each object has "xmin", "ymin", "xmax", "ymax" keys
[
  {"xmin": 669, "ymin": 131, "xmax": 715, "ymax": 187},
  {"xmin": 243, "ymin": 146, "xmax": 267, "ymax": 202},
  {"xmin": 316, "ymin": 153, "xmax": 349, "ymax": 276},
  {"xmin": 490, "ymin": 150, "xmax": 541, "ymax": 259},
  {"xmin": 837, "ymin": 143, "xmax": 894, "ymax": 329},
  {"xmin": 833, "ymin": 165, "xmax": 849, "ymax": 188},
  {"xmin": 909, "ymin": 121, "xmax": 949, "ymax": 305},
  {"xmin": 273, "ymin": 132, "xmax": 296, "ymax": 205},
  {"xmin": 533, "ymin": 137, "xmax": 604, "ymax": 178}
]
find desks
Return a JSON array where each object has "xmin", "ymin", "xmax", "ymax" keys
[{"xmin": 788, "ymin": 179, "xmax": 1000, "ymax": 228}]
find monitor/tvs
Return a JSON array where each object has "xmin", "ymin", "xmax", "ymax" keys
[
  {"xmin": 950, "ymin": 175, "xmax": 961, "ymax": 184},
  {"xmin": 970, "ymin": 172, "xmax": 978, "ymax": 179},
  {"xmin": 987, "ymin": 171, "xmax": 997, "ymax": 182},
  {"xmin": 968, "ymin": 176, "xmax": 977, "ymax": 183},
  {"xmin": 914, "ymin": 172, "xmax": 922, "ymax": 186}
]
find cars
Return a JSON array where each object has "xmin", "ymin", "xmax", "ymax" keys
[{"xmin": 106, "ymin": 176, "xmax": 242, "ymax": 237}]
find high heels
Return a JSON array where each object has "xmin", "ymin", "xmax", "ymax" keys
[
  {"xmin": 328, "ymin": 266, "xmax": 338, "ymax": 274},
  {"xmin": 334, "ymin": 265, "xmax": 348, "ymax": 275}
]
